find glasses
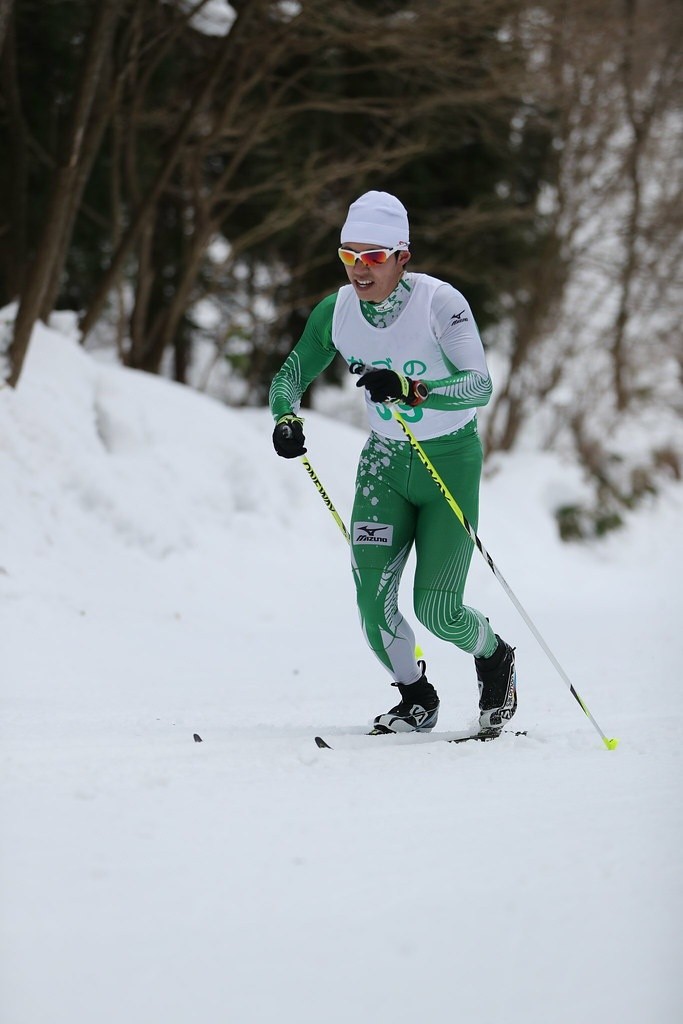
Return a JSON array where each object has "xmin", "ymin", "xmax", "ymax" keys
[{"xmin": 337, "ymin": 241, "xmax": 410, "ymax": 268}]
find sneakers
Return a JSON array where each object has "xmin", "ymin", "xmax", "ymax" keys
[
  {"xmin": 474, "ymin": 634, "xmax": 518, "ymax": 728},
  {"xmin": 373, "ymin": 660, "xmax": 441, "ymax": 735}
]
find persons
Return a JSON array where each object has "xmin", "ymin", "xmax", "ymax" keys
[{"xmin": 269, "ymin": 187, "xmax": 518, "ymax": 730}]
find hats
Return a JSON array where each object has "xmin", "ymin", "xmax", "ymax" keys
[{"xmin": 340, "ymin": 190, "xmax": 409, "ymax": 250}]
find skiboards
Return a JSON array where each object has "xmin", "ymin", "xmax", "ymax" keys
[{"xmin": 179, "ymin": 727, "xmax": 534, "ymax": 752}]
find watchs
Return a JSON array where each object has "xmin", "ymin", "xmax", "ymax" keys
[{"xmin": 410, "ymin": 380, "xmax": 429, "ymax": 408}]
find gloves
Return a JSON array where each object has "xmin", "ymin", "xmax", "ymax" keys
[
  {"xmin": 273, "ymin": 412, "xmax": 307, "ymax": 459},
  {"xmin": 356, "ymin": 368, "xmax": 418, "ymax": 406}
]
